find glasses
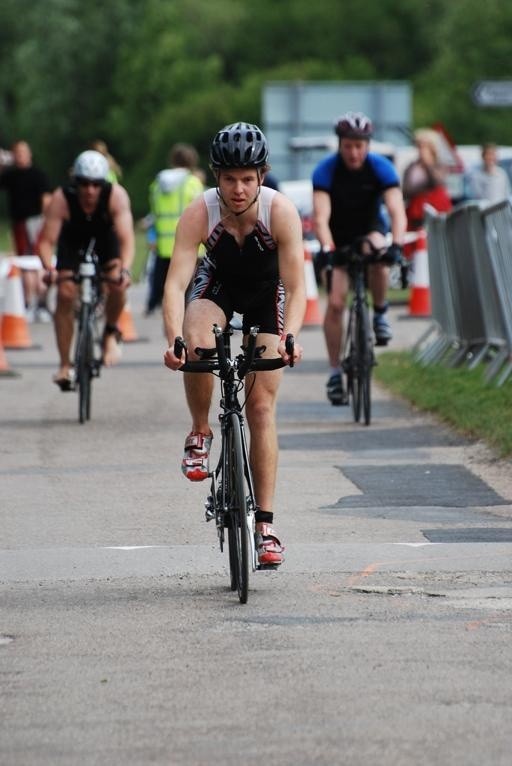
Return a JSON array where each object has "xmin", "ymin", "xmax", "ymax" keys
[{"xmin": 80, "ymin": 178, "xmax": 104, "ymax": 187}]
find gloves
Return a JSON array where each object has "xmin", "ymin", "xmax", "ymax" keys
[
  {"xmin": 314, "ymin": 250, "xmax": 339, "ymax": 271},
  {"xmin": 378, "ymin": 244, "xmax": 404, "ymax": 265}
]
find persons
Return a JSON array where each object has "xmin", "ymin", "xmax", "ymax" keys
[
  {"xmin": 401, "ymin": 128, "xmax": 446, "ymax": 288},
  {"xmin": 312, "ymin": 113, "xmax": 408, "ymax": 404},
  {"xmin": 466, "ymin": 140, "xmax": 511, "ymax": 199},
  {"xmin": 161, "ymin": 122, "xmax": 307, "ymax": 566},
  {"xmin": 0, "ymin": 140, "xmax": 53, "ymax": 324},
  {"xmin": 37, "ymin": 150, "xmax": 134, "ymax": 384},
  {"xmin": 91, "ymin": 140, "xmax": 279, "ymax": 317}
]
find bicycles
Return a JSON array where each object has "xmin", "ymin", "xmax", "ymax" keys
[
  {"xmin": 42, "ymin": 255, "xmax": 123, "ymax": 422},
  {"xmin": 314, "ymin": 238, "xmax": 408, "ymax": 425},
  {"xmin": 173, "ymin": 324, "xmax": 294, "ymax": 605}
]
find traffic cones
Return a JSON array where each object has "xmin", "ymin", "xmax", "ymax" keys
[
  {"xmin": 301, "ymin": 246, "xmax": 321, "ymax": 328},
  {"xmin": 120, "ymin": 301, "xmax": 138, "ymax": 343},
  {"xmin": 1, "ymin": 264, "xmax": 31, "ymax": 348},
  {"xmin": 404, "ymin": 229, "xmax": 430, "ymax": 319}
]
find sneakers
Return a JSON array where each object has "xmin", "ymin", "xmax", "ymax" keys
[
  {"xmin": 326, "ymin": 373, "xmax": 345, "ymax": 403},
  {"xmin": 372, "ymin": 315, "xmax": 393, "ymax": 341},
  {"xmin": 181, "ymin": 430, "xmax": 214, "ymax": 482},
  {"xmin": 253, "ymin": 524, "xmax": 285, "ymax": 564}
]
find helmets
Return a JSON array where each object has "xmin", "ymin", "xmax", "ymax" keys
[
  {"xmin": 334, "ymin": 111, "xmax": 373, "ymax": 138},
  {"xmin": 72, "ymin": 149, "xmax": 108, "ymax": 181},
  {"xmin": 208, "ymin": 121, "xmax": 269, "ymax": 171}
]
